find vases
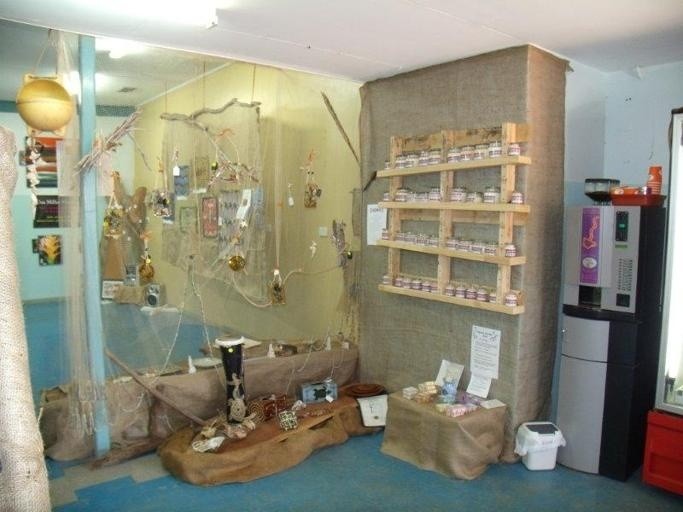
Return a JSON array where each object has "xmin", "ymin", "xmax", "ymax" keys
[{"xmin": 215, "ymin": 336, "xmax": 246, "ymax": 424}]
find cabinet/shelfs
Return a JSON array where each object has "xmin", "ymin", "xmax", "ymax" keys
[
  {"xmin": 635, "ymin": 407, "xmax": 683, "ymax": 498},
  {"xmin": 377, "ymin": 123, "xmax": 532, "ymax": 316}
]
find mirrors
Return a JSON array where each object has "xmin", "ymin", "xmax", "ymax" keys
[{"xmin": 651, "ymin": 107, "xmax": 683, "ymax": 419}]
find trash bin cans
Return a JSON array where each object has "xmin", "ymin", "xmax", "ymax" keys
[{"xmin": 514, "ymin": 421, "xmax": 566, "ymax": 471}]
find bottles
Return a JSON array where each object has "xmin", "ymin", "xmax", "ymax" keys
[
  {"xmin": 382, "ymin": 273, "xmax": 523, "ymax": 309},
  {"xmin": 378, "ymin": 142, "xmax": 521, "ymax": 170},
  {"xmin": 373, "ymin": 186, "xmax": 524, "ymax": 204},
  {"xmin": 378, "ymin": 226, "xmax": 517, "ymax": 259}
]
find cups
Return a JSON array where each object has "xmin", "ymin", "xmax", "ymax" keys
[
  {"xmin": 645, "ymin": 166, "xmax": 662, "ymax": 195},
  {"xmin": 610, "ymin": 186, "xmax": 640, "ymax": 196}
]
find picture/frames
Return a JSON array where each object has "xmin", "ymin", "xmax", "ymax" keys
[
  {"xmin": 100, "ymin": 279, "xmax": 125, "ymax": 300},
  {"xmin": 180, "ymin": 206, "xmax": 198, "ymax": 235},
  {"xmin": 203, "ymin": 197, "xmax": 218, "ymax": 238}
]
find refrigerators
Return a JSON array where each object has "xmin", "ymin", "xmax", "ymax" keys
[{"xmin": 552, "ymin": 206, "xmax": 668, "ymax": 483}]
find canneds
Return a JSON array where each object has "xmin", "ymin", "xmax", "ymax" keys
[
  {"xmin": 504, "ymin": 243, "xmax": 517, "ymax": 257},
  {"xmin": 382, "ymin": 229, "xmax": 389, "ymax": 240},
  {"xmin": 505, "ymin": 294, "xmax": 518, "ymax": 306},
  {"xmin": 394, "ymin": 148, "xmax": 441, "ymax": 169},
  {"xmin": 382, "ymin": 273, "xmax": 391, "ymax": 285},
  {"xmin": 483, "ymin": 186, "xmax": 501, "ymax": 203},
  {"xmin": 447, "ymin": 141, "xmax": 502, "ymax": 163},
  {"xmin": 394, "ymin": 186, "xmax": 441, "ymax": 202},
  {"xmin": 383, "ymin": 160, "xmax": 390, "ymax": 169},
  {"xmin": 466, "ymin": 192, "xmax": 483, "ymax": 203},
  {"xmin": 445, "ymin": 282, "xmax": 496, "ymax": 303},
  {"xmin": 449, "ymin": 187, "xmax": 468, "ymax": 201},
  {"xmin": 395, "ymin": 231, "xmax": 439, "ymax": 247},
  {"xmin": 508, "ymin": 142, "xmax": 521, "ymax": 155},
  {"xmin": 447, "ymin": 237, "xmax": 498, "ymax": 255},
  {"xmin": 395, "ymin": 275, "xmax": 438, "ymax": 293},
  {"xmin": 383, "ymin": 191, "xmax": 389, "ymax": 201},
  {"xmin": 511, "ymin": 191, "xmax": 524, "ymax": 204}
]
